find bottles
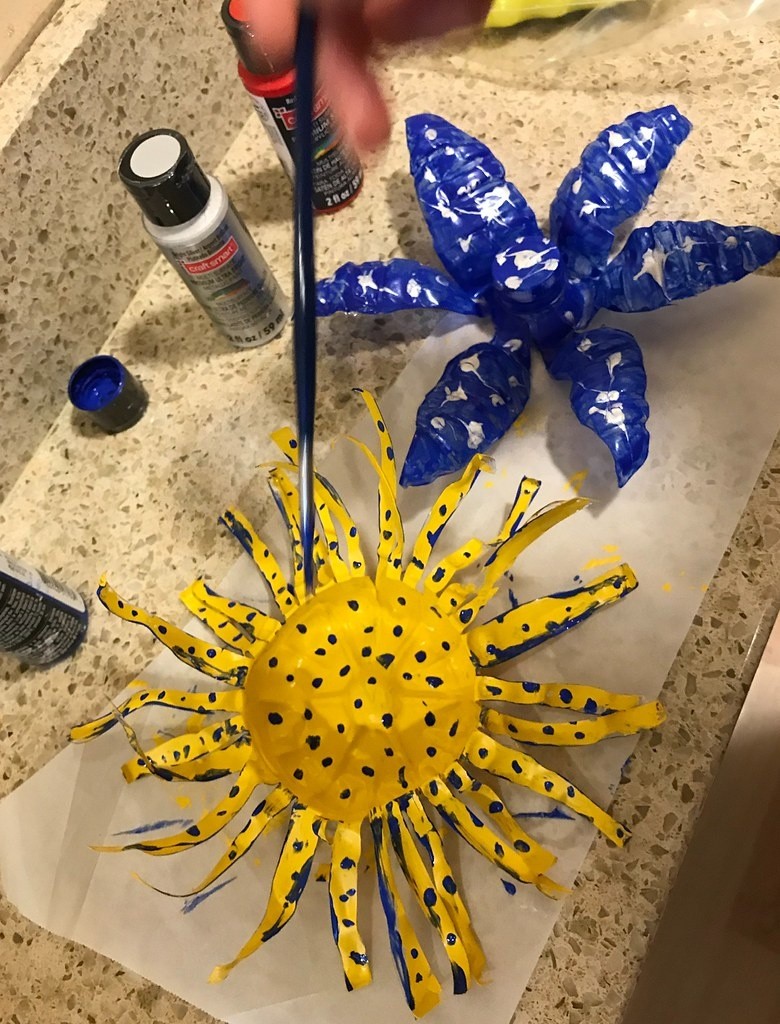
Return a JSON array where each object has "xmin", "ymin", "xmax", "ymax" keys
[
  {"xmin": 0, "ymin": 552, "xmax": 88, "ymax": 667},
  {"xmin": 222, "ymin": 0, "xmax": 366, "ymax": 215},
  {"xmin": 118, "ymin": 128, "xmax": 289, "ymax": 348}
]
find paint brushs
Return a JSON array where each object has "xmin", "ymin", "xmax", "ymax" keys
[{"xmin": 290, "ymin": 0, "xmax": 321, "ymax": 605}]
